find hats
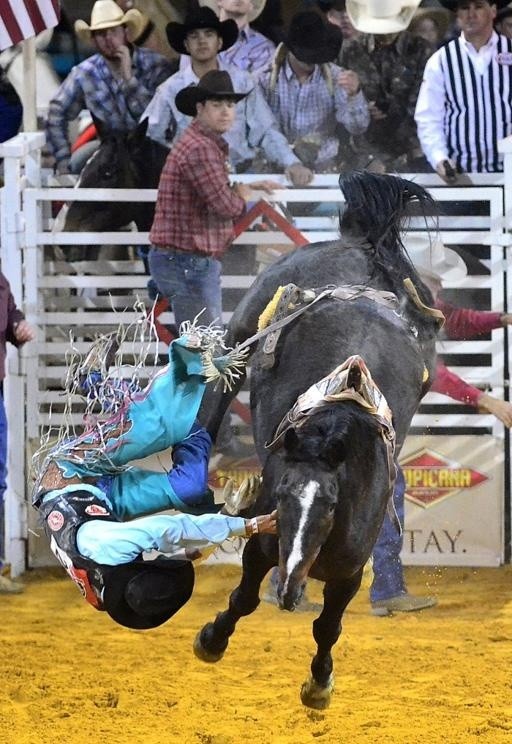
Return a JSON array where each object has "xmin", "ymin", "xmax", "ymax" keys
[
  {"xmin": 199, "ymin": 0, "xmax": 266, "ymax": 22},
  {"xmin": 175, "ymin": 70, "xmax": 253, "ymax": 117},
  {"xmin": 281, "ymin": 8, "xmax": 343, "ymax": 64},
  {"xmin": 401, "ymin": 232, "xmax": 468, "ymax": 287},
  {"xmin": 102, "ymin": 558, "xmax": 195, "ymax": 630},
  {"xmin": 74, "ymin": 0, "xmax": 144, "ymax": 47},
  {"xmin": 406, "ymin": 7, "xmax": 448, "ymax": 45},
  {"xmin": 165, "ymin": 6, "xmax": 240, "ymax": 56},
  {"xmin": 346, "ymin": 0, "xmax": 423, "ymax": 35}
]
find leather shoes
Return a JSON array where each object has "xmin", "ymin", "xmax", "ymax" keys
[
  {"xmin": 371, "ymin": 593, "xmax": 434, "ymax": 616},
  {"xmin": 264, "ymin": 580, "xmax": 324, "ymax": 613},
  {"xmin": 71, "ymin": 331, "xmax": 121, "ymax": 395}
]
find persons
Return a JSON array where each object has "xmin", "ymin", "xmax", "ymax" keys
[
  {"xmin": 28, "ymin": 332, "xmax": 277, "ymax": 630},
  {"xmin": 36, "ymin": 0, "xmax": 512, "ymax": 311},
  {"xmin": 262, "ymin": 237, "xmax": 512, "ymax": 617},
  {"xmin": 148, "ymin": 69, "xmax": 287, "ymax": 456},
  {"xmin": 0, "ymin": 65, "xmax": 24, "ymax": 142},
  {"xmin": 0, "ymin": 262, "xmax": 34, "ymax": 595}
]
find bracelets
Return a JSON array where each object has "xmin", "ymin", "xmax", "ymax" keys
[{"xmin": 250, "ymin": 519, "xmax": 259, "ymax": 535}]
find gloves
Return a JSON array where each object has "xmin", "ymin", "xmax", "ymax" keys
[{"xmin": 222, "ymin": 476, "xmax": 261, "ymax": 520}]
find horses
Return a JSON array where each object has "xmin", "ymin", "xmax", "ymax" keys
[
  {"xmin": 51, "ymin": 108, "xmax": 493, "ymax": 435},
  {"xmin": 189, "ymin": 169, "xmax": 440, "ymax": 722}
]
